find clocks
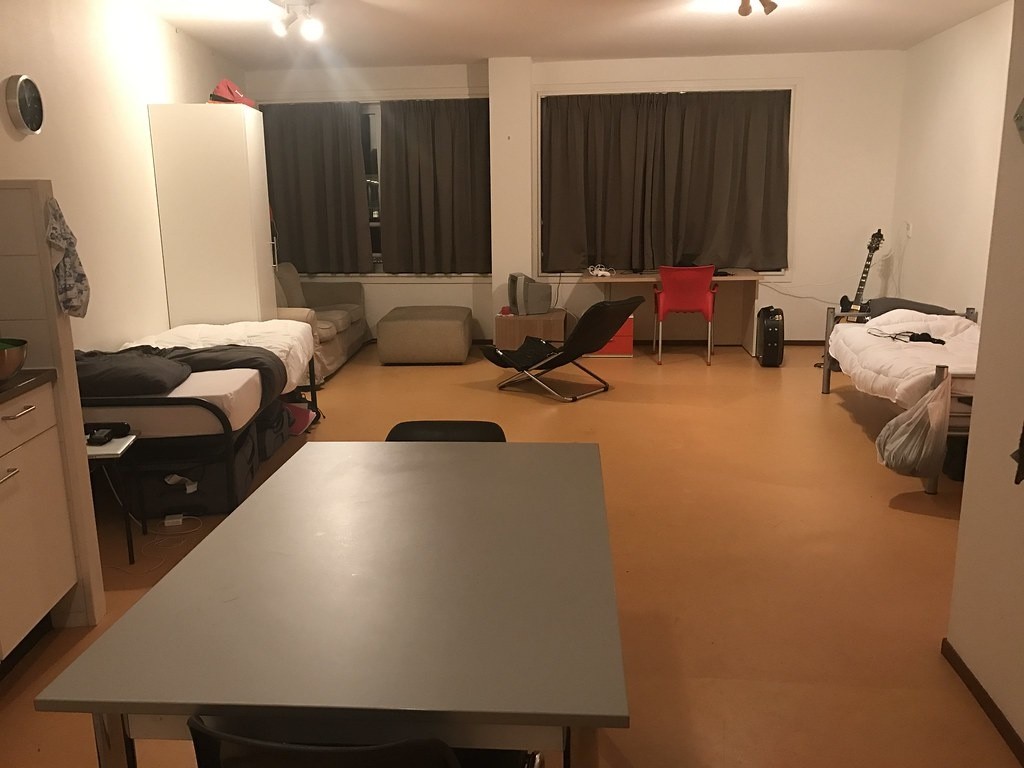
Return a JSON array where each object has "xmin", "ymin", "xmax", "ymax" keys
[{"xmin": 5, "ymin": 75, "xmax": 45, "ymax": 135}]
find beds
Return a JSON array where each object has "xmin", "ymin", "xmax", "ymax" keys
[
  {"xmin": 73, "ymin": 320, "xmax": 320, "ymax": 516},
  {"xmin": 823, "ymin": 308, "xmax": 979, "ymax": 494}
]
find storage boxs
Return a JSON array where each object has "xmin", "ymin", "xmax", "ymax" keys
[{"xmin": 582, "ymin": 314, "xmax": 634, "ymax": 359}]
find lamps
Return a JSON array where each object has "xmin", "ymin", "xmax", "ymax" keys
[
  {"xmin": 267, "ymin": 0, "xmax": 323, "ymax": 41},
  {"xmin": 738, "ymin": 0, "xmax": 778, "ymax": 15}
]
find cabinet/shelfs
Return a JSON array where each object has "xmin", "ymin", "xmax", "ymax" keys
[
  {"xmin": 148, "ymin": 100, "xmax": 279, "ymax": 321},
  {"xmin": 0, "ymin": 179, "xmax": 106, "ymax": 666}
]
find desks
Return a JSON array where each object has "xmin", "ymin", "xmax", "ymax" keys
[
  {"xmin": 578, "ymin": 271, "xmax": 763, "ymax": 364},
  {"xmin": 495, "ymin": 308, "xmax": 568, "ymax": 350},
  {"xmin": 86, "ymin": 431, "xmax": 145, "ymax": 565},
  {"xmin": 35, "ymin": 442, "xmax": 634, "ymax": 767}
]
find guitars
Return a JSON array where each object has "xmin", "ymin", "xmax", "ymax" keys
[{"xmin": 831, "ymin": 229, "xmax": 885, "ymax": 325}]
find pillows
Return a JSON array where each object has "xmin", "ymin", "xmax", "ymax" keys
[
  {"xmin": 83, "ymin": 358, "xmax": 192, "ymax": 397},
  {"xmin": 868, "ymin": 298, "xmax": 956, "ymax": 317}
]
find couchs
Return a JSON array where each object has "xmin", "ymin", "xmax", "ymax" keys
[{"xmin": 267, "ymin": 262, "xmax": 371, "ymax": 388}]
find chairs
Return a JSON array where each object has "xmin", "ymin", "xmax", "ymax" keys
[
  {"xmin": 481, "ymin": 295, "xmax": 646, "ymax": 402},
  {"xmin": 382, "ymin": 419, "xmax": 507, "ymax": 442},
  {"xmin": 652, "ymin": 265, "xmax": 717, "ymax": 365},
  {"xmin": 188, "ymin": 717, "xmax": 545, "ymax": 768}
]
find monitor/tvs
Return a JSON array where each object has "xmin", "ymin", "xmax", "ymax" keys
[{"xmin": 508, "ymin": 271, "xmax": 565, "ymax": 317}]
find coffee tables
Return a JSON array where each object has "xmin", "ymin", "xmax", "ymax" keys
[{"xmin": 378, "ymin": 305, "xmax": 474, "ymax": 364}]
